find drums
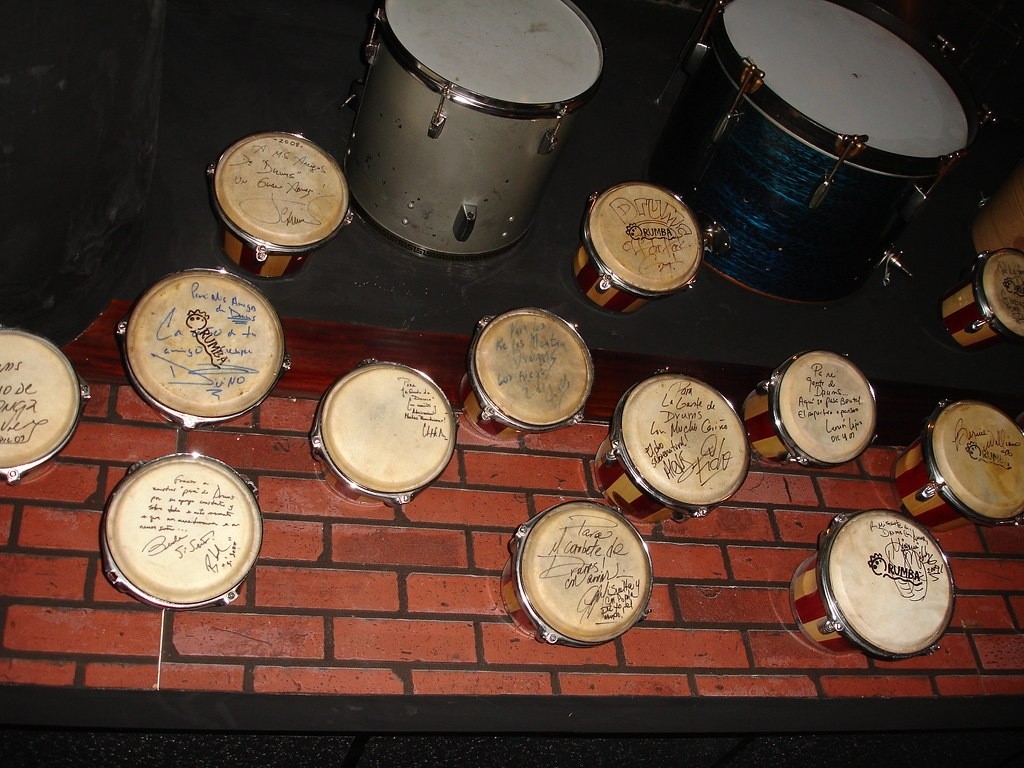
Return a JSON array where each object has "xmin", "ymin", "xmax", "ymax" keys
[
  {"xmin": 789, "ymin": 506, "xmax": 957, "ymax": 663},
  {"xmin": 571, "ymin": 181, "xmax": 706, "ymax": 318},
  {"xmin": 307, "ymin": 356, "xmax": 465, "ymax": 506},
  {"xmin": 99, "ymin": 452, "xmax": 265, "ymax": 612},
  {"xmin": 968, "ymin": 163, "xmax": 1024, "ymax": 254},
  {"xmin": 889, "ymin": 394, "xmax": 1023, "ymax": 533},
  {"xmin": 742, "ymin": 349, "xmax": 880, "ymax": 469},
  {"xmin": 1, "ymin": 327, "xmax": 90, "ymax": 486},
  {"xmin": 459, "ymin": 306, "xmax": 595, "ymax": 442},
  {"xmin": 500, "ymin": 499, "xmax": 657, "ymax": 649},
  {"xmin": 593, "ymin": 366, "xmax": 751, "ymax": 525},
  {"xmin": 342, "ymin": 0, "xmax": 604, "ymax": 269},
  {"xmin": 643, "ymin": 2, "xmax": 997, "ymax": 307},
  {"xmin": 205, "ymin": 128, "xmax": 355, "ymax": 279},
  {"xmin": 114, "ymin": 266, "xmax": 293, "ymax": 432},
  {"xmin": 940, "ymin": 248, "xmax": 1024, "ymax": 348}
]
